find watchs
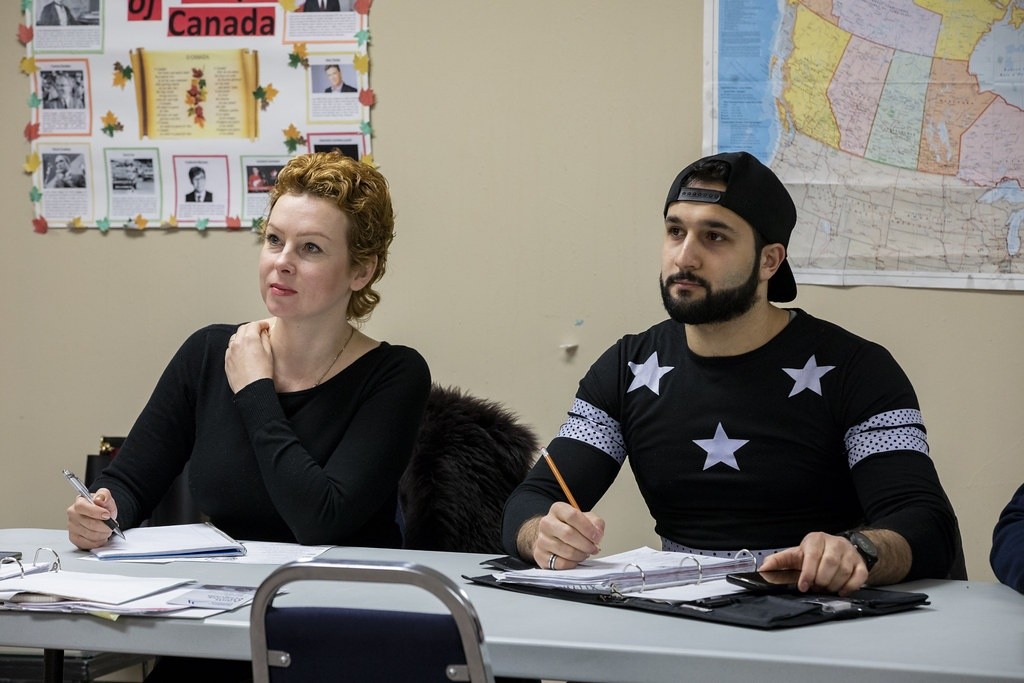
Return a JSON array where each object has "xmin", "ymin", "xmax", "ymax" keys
[{"xmin": 835, "ymin": 529, "xmax": 880, "ymax": 572}]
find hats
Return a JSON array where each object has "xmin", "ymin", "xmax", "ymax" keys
[{"xmin": 661, "ymin": 150, "xmax": 798, "ymax": 304}]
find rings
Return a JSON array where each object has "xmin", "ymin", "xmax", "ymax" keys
[{"xmin": 547, "ymin": 552, "xmax": 559, "ymax": 569}]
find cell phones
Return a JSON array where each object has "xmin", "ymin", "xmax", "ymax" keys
[{"xmin": 727, "ymin": 569, "xmax": 802, "ymax": 592}]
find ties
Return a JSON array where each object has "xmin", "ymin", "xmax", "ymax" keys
[
  {"xmin": 321, "ymin": 0, "xmax": 324, "ymax": 11},
  {"xmin": 198, "ymin": 196, "xmax": 201, "ymax": 202}
]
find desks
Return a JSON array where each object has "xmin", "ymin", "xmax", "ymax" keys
[{"xmin": 0, "ymin": 529, "xmax": 1024, "ymax": 683}]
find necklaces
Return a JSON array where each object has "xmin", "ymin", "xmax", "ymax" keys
[{"xmin": 312, "ymin": 324, "xmax": 355, "ymax": 390}]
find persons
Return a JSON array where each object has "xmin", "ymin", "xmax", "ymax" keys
[
  {"xmin": 182, "ymin": 165, "xmax": 216, "ymax": 207},
  {"xmin": 315, "ymin": 64, "xmax": 356, "ymax": 96},
  {"xmin": 65, "ymin": 152, "xmax": 430, "ymax": 556},
  {"xmin": 504, "ymin": 148, "xmax": 952, "ymax": 595},
  {"xmin": 990, "ymin": 479, "xmax": 1024, "ymax": 601}
]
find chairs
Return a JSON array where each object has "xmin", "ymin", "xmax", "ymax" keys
[{"xmin": 249, "ymin": 559, "xmax": 495, "ymax": 683}]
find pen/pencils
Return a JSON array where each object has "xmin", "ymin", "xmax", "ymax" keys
[
  {"xmin": 62, "ymin": 468, "xmax": 126, "ymax": 541},
  {"xmin": 539, "ymin": 446, "xmax": 603, "ymax": 550}
]
